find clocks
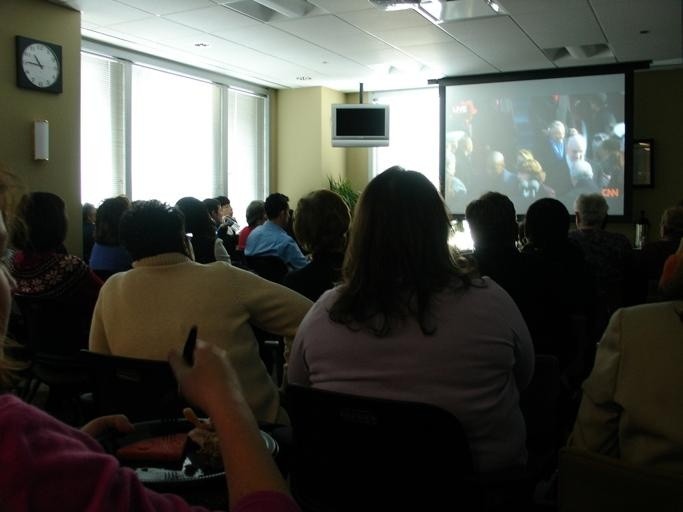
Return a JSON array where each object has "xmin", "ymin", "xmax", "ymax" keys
[{"xmin": 14, "ymin": 34, "xmax": 63, "ymax": 94}]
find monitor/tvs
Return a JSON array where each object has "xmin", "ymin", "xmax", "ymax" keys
[{"xmin": 330, "ymin": 104, "xmax": 389, "ymax": 148}]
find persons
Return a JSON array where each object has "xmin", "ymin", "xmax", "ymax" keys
[
  {"xmin": 283, "ymin": 188, "xmax": 351, "ymax": 303},
  {"xmin": 244, "ymin": 193, "xmax": 313, "ymax": 273},
  {"xmin": 88, "ymin": 195, "xmax": 133, "ymax": 270},
  {"xmin": 174, "ymin": 197, "xmax": 232, "ymax": 265},
  {"xmin": 0, "ymin": 193, "xmax": 111, "ymax": 420},
  {"xmin": 236, "ymin": 200, "xmax": 268, "ymax": 251},
  {"xmin": 215, "ymin": 196, "xmax": 242, "ymax": 245},
  {"xmin": 570, "ymin": 192, "xmax": 634, "ymax": 276},
  {"xmin": 203, "ymin": 199, "xmax": 222, "ymax": 231},
  {"xmin": 457, "ymin": 191, "xmax": 563, "ymax": 362},
  {"xmin": 287, "ymin": 166, "xmax": 534, "ymax": 512},
  {"xmin": 642, "ymin": 207, "xmax": 683, "ymax": 303},
  {"xmin": 0, "ymin": 167, "xmax": 299, "ymax": 512},
  {"xmin": 522, "ymin": 198, "xmax": 572, "ymax": 268},
  {"xmin": 89, "ymin": 199, "xmax": 314, "ymax": 442},
  {"xmin": 560, "ymin": 238, "xmax": 683, "ymax": 512},
  {"xmin": 82, "ymin": 203, "xmax": 97, "ymax": 256},
  {"xmin": 444, "ymin": 93, "xmax": 625, "ymax": 215}
]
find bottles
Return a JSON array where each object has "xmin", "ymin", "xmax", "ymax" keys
[
  {"xmin": 222, "ymin": 216, "xmax": 240, "ymax": 232},
  {"xmin": 633, "ymin": 209, "xmax": 651, "ymax": 250}
]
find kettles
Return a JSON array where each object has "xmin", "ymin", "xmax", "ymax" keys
[{"xmin": 635, "ymin": 218, "xmax": 649, "ymax": 247}]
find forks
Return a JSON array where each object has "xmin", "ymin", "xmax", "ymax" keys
[{"xmin": 164, "ymin": 326, "xmax": 199, "ymax": 444}]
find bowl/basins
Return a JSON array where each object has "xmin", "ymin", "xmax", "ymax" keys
[{"xmin": 89, "ymin": 419, "xmax": 280, "ymax": 500}]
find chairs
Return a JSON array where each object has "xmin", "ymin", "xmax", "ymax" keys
[
  {"xmin": 10, "ymin": 291, "xmax": 87, "ymax": 419},
  {"xmin": 75, "ymin": 350, "xmax": 180, "ymax": 420},
  {"xmin": 239, "ymin": 253, "xmax": 286, "ymax": 283},
  {"xmin": 557, "ymin": 447, "xmax": 682, "ymax": 512},
  {"xmin": 278, "ymin": 385, "xmax": 483, "ymax": 512}
]
